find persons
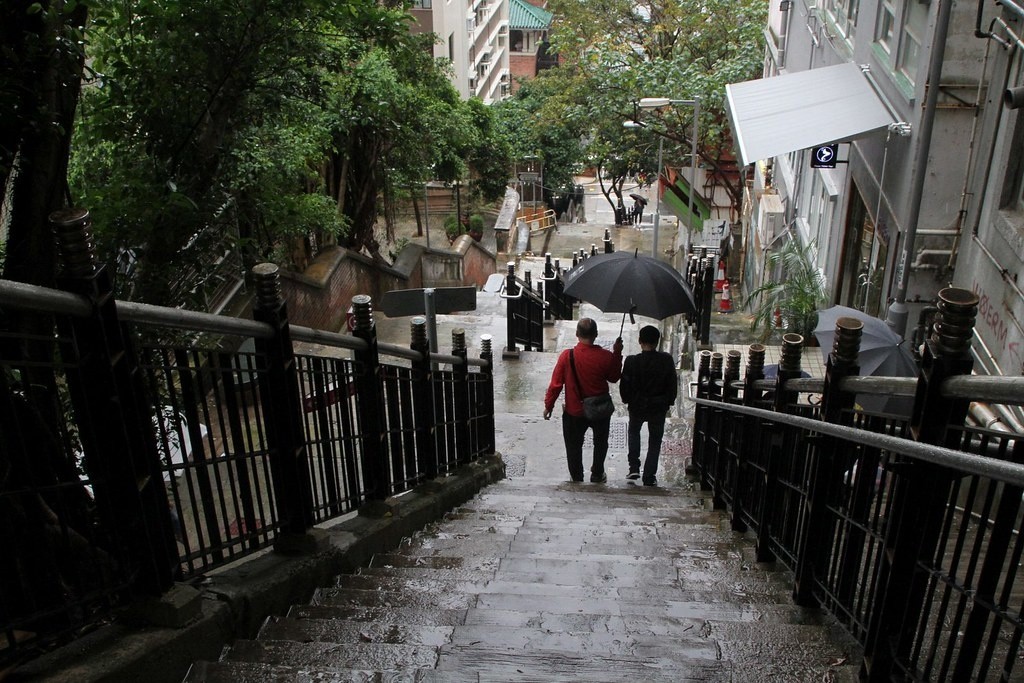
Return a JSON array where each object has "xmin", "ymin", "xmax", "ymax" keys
[
  {"xmin": 617, "ymin": 326, "xmax": 677, "ymax": 486},
  {"xmin": 544, "ymin": 318, "xmax": 623, "ymax": 482},
  {"xmin": 635, "ymin": 198, "xmax": 644, "ymax": 223}
]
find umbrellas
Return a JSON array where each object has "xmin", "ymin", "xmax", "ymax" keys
[
  {"xmin": 629, "ymin": 193, "xmax": 647, "ymax": 205},
  {"xmin": 807, "ymin": 303, "xmax": 920, "ymax": 416},
  {"xmin": 558, "ymin": 246, "xmax": 695, "ymax": 339},
  {"xmin": 762, "ymin": 363, "xmax": 811, "ymax": 380}
]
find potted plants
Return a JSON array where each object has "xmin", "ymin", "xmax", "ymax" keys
[
  {"xmin": 445, "ymin": 221, "xmax": 466, "ymax": 246},
  {"xmin": 743, "ymin": 235, "xmax": 827, "ymax": 346},
  {"xmin": 468, "ymin": 214, "xmax": 484, "ymax": 242}
]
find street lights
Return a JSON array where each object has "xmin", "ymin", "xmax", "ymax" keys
[
  {"xmin": 524, "ymin": 154, "xmax": 542, "ymax": 222},
  {"xmin": 624, "ymin": 120, "xmax": 666, "ymax": 262},
  {"xmin": 638, "ymin": 94, "xmax": 701, "ymax": 280}
]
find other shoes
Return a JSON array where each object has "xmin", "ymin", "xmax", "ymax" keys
[
  {"xmin": 643, "ymin": 479, "xmax": 657, "ymax": 486},
  {"xmin": 591, "ymin": 473, "xmax": 607, "ymax": 482},
  {"xmin": 626, "ymin": 470, "xmax": 640, "ymax": 479}
]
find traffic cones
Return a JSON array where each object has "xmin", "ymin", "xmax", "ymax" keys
[
  {"xmin": 770, "ymin": 309, "xmax": 785, "ymax": 331},
  {"xmin": 714, "ymin": 260, "xmax": 727, "ymax": 293},
  {"xmin": 717, "ymin": 280, "xmax": 734, "ymax": 313}
]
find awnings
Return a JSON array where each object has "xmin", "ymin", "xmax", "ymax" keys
[{"xmin": 725, "ymin": 60, "xmax": 894, "ymax": 175}]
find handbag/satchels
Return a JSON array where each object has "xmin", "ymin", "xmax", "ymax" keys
[{"xmin": 582, "ymin": 393, "xmax": 614, "ymax": 420}]
